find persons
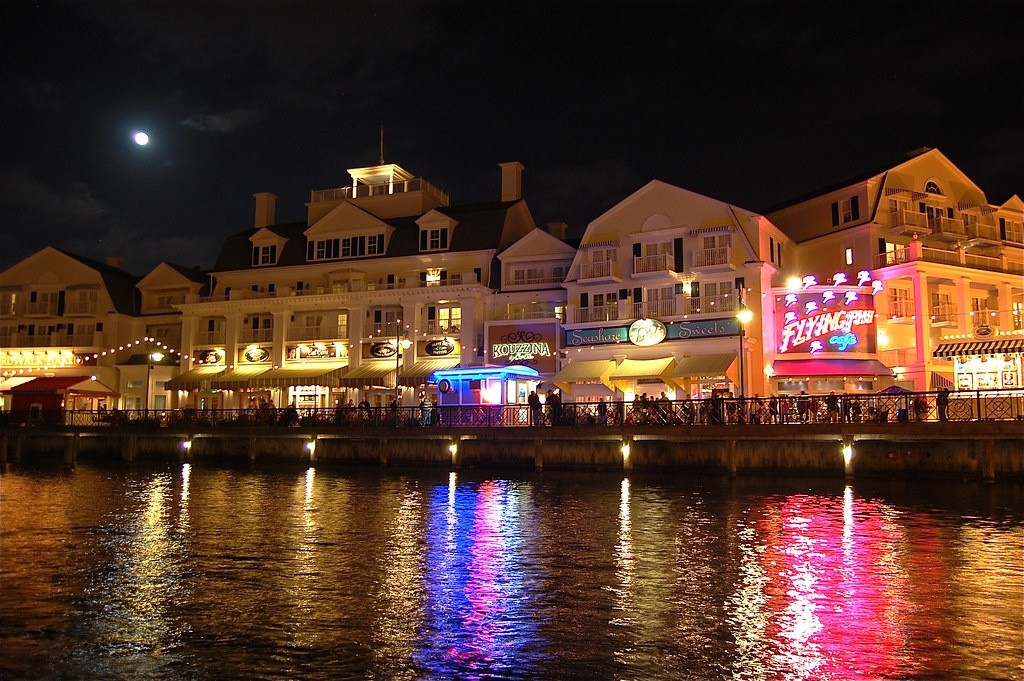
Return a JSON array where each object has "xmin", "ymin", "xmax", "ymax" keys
[
  {"xmin": 247, "ymin": 395, "xmax": 278, "ymax": 422},
  {"xmin": 935, "ymin": 387, "xmax": 951, "ymax": 422},
  {"xmin": 683, "ymin": 393, "xmax": 695, "ymax": 425},
  {"xmin": 727, "ymin": 391, "xmax": 863, "ymax": 423},
  {"xmin": 345, "ymin": 399, "xmax": 357, "ymax": 421},
  {"xmin": 546, "ymin": 389, "xmax": 563, "ymax": 428},
  {"xmin": 528, "ymin": 391, "xmax": 544, "ymax": 426},
  {"xmin": 597, "ymin": 398, "xmax": 608, "ymax": 426},
  {"xmin": 358, "ymin": 396, "xmax": 372, "ymax": 421},
  {"xmin": 633, "ymin": 391, "xmax": 670, "ymax": 424}
]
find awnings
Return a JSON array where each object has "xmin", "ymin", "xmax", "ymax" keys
[
  {"xmin": 551, "ymin": 352, "xmax": 740, "ymax": 394},
  {"xmin": 164, "ymin": 364, "xmax": 227, "ymax": 394},
  {"xmin": 932, "ymin": 339, "xmax": 1024, "ymax": 357},
  {"xmin": 209, "ymin": 360, "xmax": 273, "ymax": 390},
  {"xmin": 772, "ymin": 360, "xmax": 893, "ymax": 377},
  {"xmin": 397, "ymin": 359, "xmax": 460, "ymax": 387},
  {"xmin": 339, "ymin": 360, "xmax": 400, "ymax": 389},
  {"xmin": 249, "ymin": 361, "xmax": 348, "ymax": 388}
]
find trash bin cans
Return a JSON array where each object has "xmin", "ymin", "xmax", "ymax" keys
[{"xmin": 897, "ymin": 409, "xmax": 909, "ymax": 422}]
